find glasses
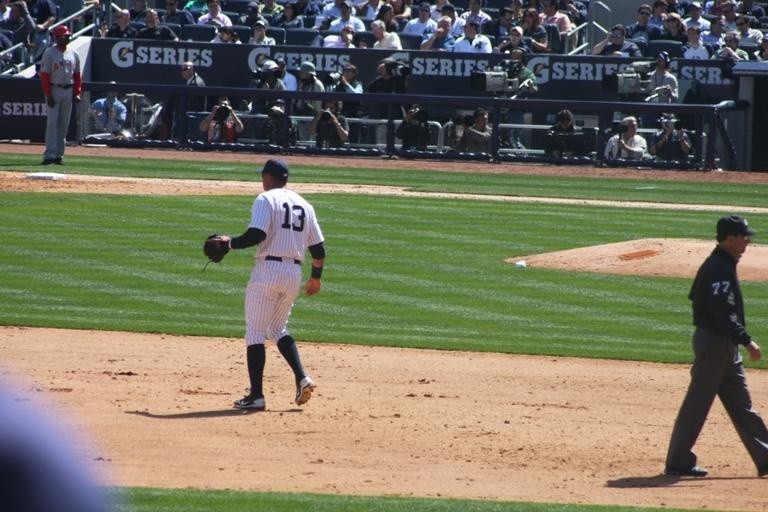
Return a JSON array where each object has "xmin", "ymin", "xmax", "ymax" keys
[{"xmin": 609, "ymin": 7, "xmax": 754, "ymax": 49}]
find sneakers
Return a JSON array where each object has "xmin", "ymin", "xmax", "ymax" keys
[
  {"xmin": 294, "ymin": 375, "xmax": 314, "ymax": 405},
  {"xmin": 664, "ymin": 464, "xmax": 708, "ymax": 477},
  {"xmin": 40, "ymin": 157, "xmax": 63, "ymax": 165},
  {"xmin": 233, "ymin": 394, "xmax": 266, "ymax": 409}
]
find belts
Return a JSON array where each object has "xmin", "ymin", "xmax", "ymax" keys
[
  {"xmin": 265, "ymin": 256, "xmax": 300, "ymax": 264},
  {"xmin": 50, "ymin": 83, "xmax": 73, "ymax": 89}
]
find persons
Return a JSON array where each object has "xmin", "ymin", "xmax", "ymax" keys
[
  {"xmin": 664, "ymin": 214, "xmax": 768, "ymax": 478},
  {"xmin": 204, "ymin": 158, "xmax": 326, "ymax": 408},
  {"xmin": 1, "ymin": 1, "xmax": 767, "ymax": 173}
]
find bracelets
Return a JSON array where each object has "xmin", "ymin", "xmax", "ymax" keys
[{"xmin": 309, "ymin": 265, "xmax": 322, "ymax": 279}]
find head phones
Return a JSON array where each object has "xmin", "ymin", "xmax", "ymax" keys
[
  {"xmin": 509, "ymin": 47, "xmax": 529, "ymax": 63},
  {"xmin": 658, "ymin": 51, "xmax": 672, "ymax": 69}
]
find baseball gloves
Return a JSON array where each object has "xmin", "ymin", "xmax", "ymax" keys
[{"xmin": 204, "ymin": 233, "xmax": 231, "ymax": 263}]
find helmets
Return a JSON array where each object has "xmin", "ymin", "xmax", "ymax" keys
[{"xmin": 54, "ymin": 25, "xmax": 71, "ymax": 36}]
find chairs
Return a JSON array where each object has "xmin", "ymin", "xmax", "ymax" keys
[{"xmin": 0, "ymin": 0, "xmax": 768, "ymax": 61}]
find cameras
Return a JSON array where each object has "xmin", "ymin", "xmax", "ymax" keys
[
  {"xmin": 321, "ymin": 111, "xmax": 330, "ymax": 121},
  {"xmin": 463, "ymin": 116, "xmax": 475, "ymax": 127},
  {"xmin": 611, "ymin": 122, "xmax": 626, "ymax": 134}
]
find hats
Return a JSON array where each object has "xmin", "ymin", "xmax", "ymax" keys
[
  {"xmin": 263, "ymin": 58, "xmax": 286, "ymax": 70},
  {"xmin": 272, "ymin": 99, "xmax": 286, "ymax": 105},
  {"xmin": 252, "ymin": 20, "xmax": 266, "ymax": 30},
  {"xmin": 510, "ymin": 25, "xmax": 523, "ymax": 37},
  {"xmin": 296, "ymin": 61, "xmax": 317, "ymax": 75},
  {"xmin": 255, "ymin": 158, "xmax": 288, "ymax": 180},
  {"xmin": 716, "ymin": 214, "xmax": 758, "ymax": 236}
]
